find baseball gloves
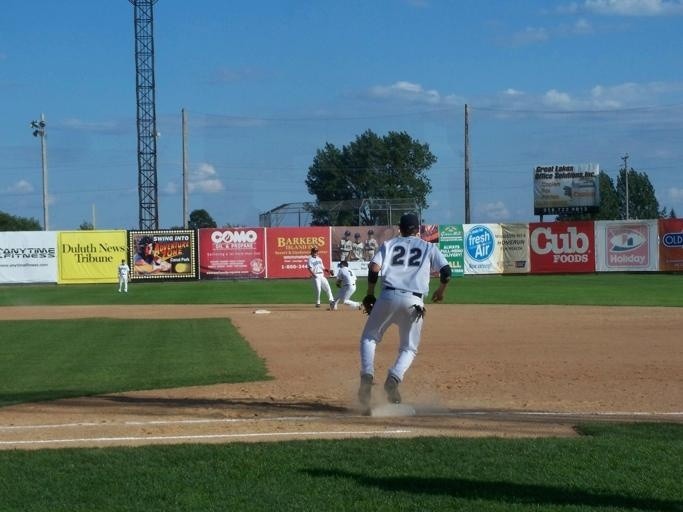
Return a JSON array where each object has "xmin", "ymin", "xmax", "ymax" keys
[
  {"xmin": 362, "ymin": 295, "xmax": 376, "ymax": 315},
  {"xmin": 336, "ymin": 280, "xmax": 342, "ymax": 288}
]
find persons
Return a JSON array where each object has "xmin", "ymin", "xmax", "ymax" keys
[
  {"xmin": 337, "ymin": 230, "xmax": 352, "ymax": 261},
  {"xmin": 351, "ymin": 232, "xmax": 364, "ymax": 260},
  {"xmin": 329, "ymin": 260, "xmax": 361, "ymax": 310},
  {"xmin": 363, "ymin": 229, "xmax": 379, "ymax": 261},
  {"xmin": 307, "ymin": 248, "xmax": 334, "ymax": 307},
  {"xmin": 116, "ymin": 259, "xmax": 130, "ymax": 293},
  {"xmin": 358, "ymin": 213, "xmax": 451, "ymax": 405},
  {"xmin": 133, "ymin": 235, "xmax": 171, "ymax": 275}
]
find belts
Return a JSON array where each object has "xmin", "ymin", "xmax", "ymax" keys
[{"xmin": 385, "ymin": 286, "xmax": 422, "ymax": 299}]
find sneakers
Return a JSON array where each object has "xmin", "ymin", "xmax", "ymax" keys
[
  {"xmin": 384, "ymin": 376, "xmax": 401, "ymax": 405},
  {"xmin": 357, "ymin": 374, "xmax": 373, "ymax": 406}
]
